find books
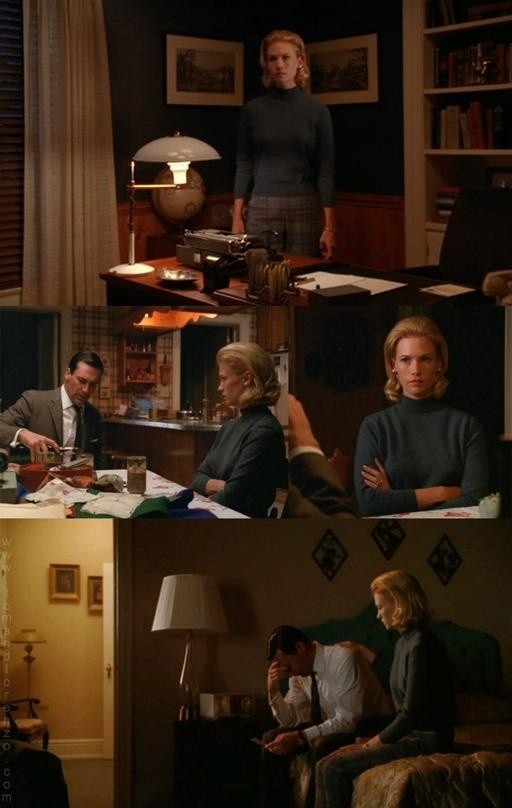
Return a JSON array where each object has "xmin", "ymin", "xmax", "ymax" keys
[
  {"xmin": 426, "ymin": 0, "xmax": 512, "ymax": 27},
  {"xmin": 434, "ymin": 42, "xmax": 510, "ymax": 88},
  {"xmin": 431, "ymin": 186, "xmax": 462, "ymax": 222},
  {"xmin": 430, "ymin": 101, "xmax": 512, "ymax": 149}
]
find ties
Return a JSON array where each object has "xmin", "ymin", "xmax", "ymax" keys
[
  {"xmin": 309, "ymin": 671, "xmax": 322, "ymax": 726},
  {"xmin": 70, "ymin": 404, "xmax": 84, "ymax": 461}
]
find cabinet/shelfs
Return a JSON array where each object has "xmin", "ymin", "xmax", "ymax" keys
[
  {"xmin": 117, "ymin": 333, "xmax": 159, "ymax": 393},
  {"xmin": 147, "ymin": 225, "xmax": 227, "ymax": 260},
  {"xmin": 404, "ymin": 1, "xmax": 511, "ymax": 269}
]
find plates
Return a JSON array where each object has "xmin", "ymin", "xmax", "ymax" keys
[{"xmin": 158, "ymin": 269, "xmax": 199, "ymax": 289}]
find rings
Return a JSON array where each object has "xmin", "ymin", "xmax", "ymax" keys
[{"xmin": 377, "ymin": 481, "xmax": 383, "ymax": 488}]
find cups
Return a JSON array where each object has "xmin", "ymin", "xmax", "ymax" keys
[
  {"xmin": 126, "ymin": 457, "xmax": 146, "ymax": 495},
  {"xmin": 77, "ymin": 454, "xmax": 93, "ymax": 467},
  {"xmin": 200, "ymin": 693, "xmax": 214, "ymax": 717}
]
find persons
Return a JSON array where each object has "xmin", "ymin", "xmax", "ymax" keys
[
  {"xmin": 261, "ymin": 626, "xmax": 396, "ymax": 808},
  {"xmin": 191, "ymin": 342, "xmax": 285, "ymax": 517},
  {"xmin": 288, "ymin": 394, "xmax": 358, "ymax": 521},
  {"xmin": 0, "ymin": 351, "xmax": 106, "ymax": 472},
  {"xmin": 354, "ymin": 315, "xmax": 489, "ymax": 516},
  {"xmin": 232, "ymin": 31, "xmax": 338, "ymax": 259},
  {"xmin": 316, "ymin": 570, "xmax": 456, "ymax": 808}
]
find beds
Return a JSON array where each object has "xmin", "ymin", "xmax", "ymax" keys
[
  {"xmin": 273, "ymin": 601, "xmax": 512, "ymax": 808},
  {"xmin": 103, "ymin": 419, "xmax": 223, "ymax": 486}
]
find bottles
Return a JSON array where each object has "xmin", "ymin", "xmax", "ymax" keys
[{"xmin": 6, "ymin": 444, "xmax": 80, "ymax": 466}]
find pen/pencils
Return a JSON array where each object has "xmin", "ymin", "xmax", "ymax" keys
[{"xmin": 250, "ymin": 737, "xmax": 267, "ymax": 750}]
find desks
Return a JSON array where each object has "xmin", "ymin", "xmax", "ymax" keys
[
  {"xmin": 100, "ymin": 252, "xmax": 477, "ymax": 307},
  {"xmin": 0, "ymin": 468, "xmax": 251, "ymax": 520}
]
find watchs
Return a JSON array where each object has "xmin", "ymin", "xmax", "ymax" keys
[
  {"xmin": 361, "ymin": 744, "xmax": 368, "ymax": 750},
  {"xmin": 298, "ymin": 731, "xmax": 305, "ymax": 746},
  {"xmin": 325, "ymin": 224, "xmax": 337, "ymax": 232}
]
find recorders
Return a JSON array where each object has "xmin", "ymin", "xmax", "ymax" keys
[{"xmin": 200, "ymin": 691, "xmax": 256, "ymax": 717}]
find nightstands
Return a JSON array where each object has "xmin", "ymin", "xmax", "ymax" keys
[{"xmin": 169, "ymin": 716, "xmax": 265, "ymax": 808}]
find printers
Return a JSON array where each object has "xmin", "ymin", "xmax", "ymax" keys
[{"xmin": 176, "ymin": 227, "xmax": 285, "ymax": 270}]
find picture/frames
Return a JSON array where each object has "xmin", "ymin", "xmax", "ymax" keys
[
  {"xmin": 49, "ymin": 564, "xmax": 82, "ymax": 603},
  {"xmin": 166, "ymin": 36, "xmax": 244, "ymax": 107},
  {"xmin": 302, "ymin": 35, "xmax": 379, "ymax": 103},
  {"xmin": 88, "ymin": 574, "xmax": 103, "ymax": 612}
]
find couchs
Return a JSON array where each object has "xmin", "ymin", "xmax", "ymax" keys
[{"xmin": 0, "ymin": 739, "xmax": 70, "ymax": 808}]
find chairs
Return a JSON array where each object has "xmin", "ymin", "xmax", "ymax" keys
[{"xmin": 0, "ymin": 698, "xmax": 49, "ymax": 752}]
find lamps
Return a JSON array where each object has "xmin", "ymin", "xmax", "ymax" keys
[
  {"xmin": 10, "ymin": 628, "xmax": 46, "ymax": 717},
  {"xmin": 150, "ymin": 574, "xmax": 230, "ymax": 732},
  {"xmin": 133, "ymin": 310, "xmax": 218, "ymax": 329},
  {"xmin": 109, "ymin": 130, "xmax": 222, "ymax": 274}
]
